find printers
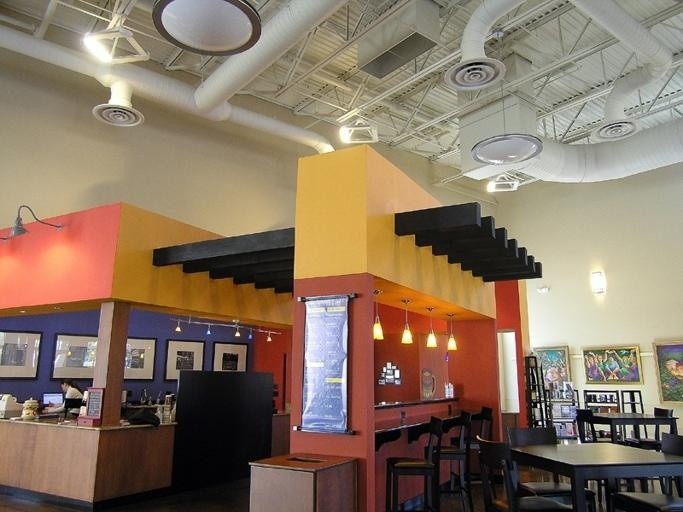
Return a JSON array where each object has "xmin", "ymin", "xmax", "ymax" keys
[{"xmin": 0, "ymin": 394, "xmax": 25, "ymax": 419}]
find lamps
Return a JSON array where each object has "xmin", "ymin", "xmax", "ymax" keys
[
  {"xmin": 372, "ymin": 288, "xmax": 384, "ymax": 340},
  {"xmin": 235, "ymin": 325, "xmax": 240, "ymax": 336},
  {"xmin": 447, "ymin": 313, "xmax": 457, "ymax": 350},
  {"xmin": 206, "ymin": 322, "xmax": 211, "ymax": 334},
  {"xmin": 400, "ymin": 298, "xmax": 413, "ymax": 344},
  {"xmin": 486, "ymin": 174, "xmax": 519, "ymax": 192},
  {"xmin": 339, "ymin": 113, "xmax": 378, "ymax": 143},
  {"xmin": 426, "ymin": 307, "xmax": 437, "ymax": 347},
  {"xmin": 175, "ymin": 319, "xmax": 181, "ymax": 331},
  {"xmin": 9, "ymin": 205, "xmax": 61, "ymax": 237},
  {"xmin": 83, "ymin": 13, "xmax": 149, "ymax": 65},
  {"xmin": 267, "ymin": 330, "xmax": 272, "ymax": 341}
]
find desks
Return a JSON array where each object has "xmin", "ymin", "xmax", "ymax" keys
[
  {"xmin": 510, "ymin": 443, "xmax": 683, "ymax": 512},
  {"xmin": 593, "ymin": 413, "xmax": 679, "ymax": 492}
]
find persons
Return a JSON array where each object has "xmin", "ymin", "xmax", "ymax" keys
[
  {"xmin": 42, "ymin": 378, "xmax": 83, "ymax": 417},
  {"xmin": 584, "ymin": 349, "xmax": 638, "ymax": 383}
]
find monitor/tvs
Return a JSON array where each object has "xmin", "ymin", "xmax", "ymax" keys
[{"xmin": 42, "ymin": 392, "xmax": 64, "ymax": 405}]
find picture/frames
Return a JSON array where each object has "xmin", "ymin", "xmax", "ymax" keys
[
  {"xmin": 0, "ymin": 329, "xmax": 44, "ymax": 381},
  {"xmin": 581, "ymin": 345, "xmax": 644, "ymax": 385},
  {"xmin": 652, "ymin": 340, "xmax": 683, "ymax": 407},
  {"xmin": 124, "ymin": 336, "xmax": 158, "ymax": 382},
  {"xmin": 164, "ymin": 338, "xmax": 206, "ymax": 381},
  {"xmin": 49, "ymin": 332, "xmax": 98, "ymax": 381},
  {"xmin": 211, "ymin": 341, "xmax": 249, "ymax": 372},
  {"xmin": 532, "ymin": 346, "xmax": 572, "ymax": 403}
]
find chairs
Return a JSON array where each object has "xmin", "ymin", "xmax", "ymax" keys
[
  {"xmin": 576, "ymin": 409, "xmax": 625, "ymax": 504},
  {"xmin": 64, "ymin": 398, "xmax": 86, "ymax": 418},
  {"xmin": 424, "ymin": 410, "xmax": 471, "ymax": 512},
  {"xmin": 611, "ymin": 432, "xmax": 683, "ymax": 512},
  {"xmin": 506, "ymin": 424, "xmax": 596, "ymax": 512},
  {"xmin": 624, "ymin": 407, "xmax": 673, "ymax": 492},
  {"xmin": 479, "ymin": 440, "xmax": 573, "ymax": 512},
  {"xmin": 450, "ymin": 406, "xmax": 493, "ymax": 496},
  {"xmin": 386, "ymin": 416, "xmax": 444, "ymax": 512}
]
